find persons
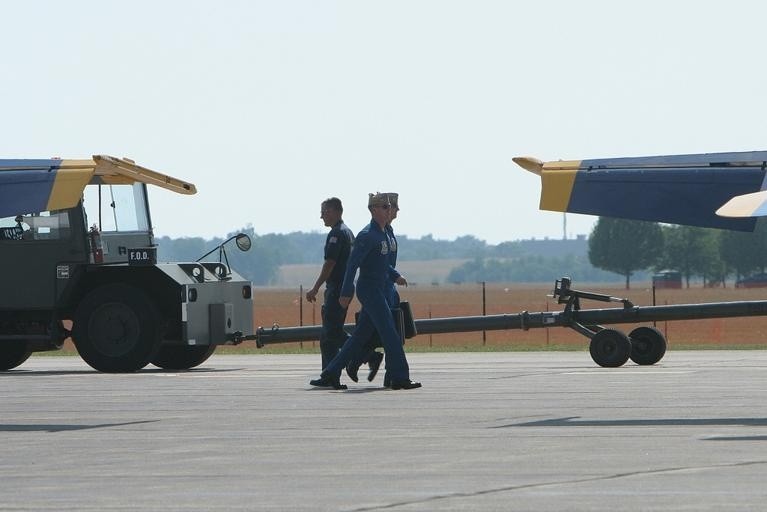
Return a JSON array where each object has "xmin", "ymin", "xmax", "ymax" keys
[
  {"xmin": 309, "ymin": 194, "xmax": 422, "ymax": 392},
  {"xmin": 345, "ymin": 191, "xmax": 402, "ymax": 389},
  {"xmin": 304, "ymin": 196, "xmax": 384, "ymax": 387}
]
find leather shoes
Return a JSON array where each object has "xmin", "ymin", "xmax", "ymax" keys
[
  {"xmin": 309, "ymin": 375, "xmax": 348, "ymax": 389},
  {"xmin": 345, "ymin": 358, "xmax": 362, "ymax": 382},
  {"xmin": 366, "ymin": 350, "xmax": 385, "ymax": 383},
  {"xmin": 383, "ymin": 373, "xmax": 423, "ymax": 391}
]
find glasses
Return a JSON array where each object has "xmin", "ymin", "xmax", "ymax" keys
[{"xmin": 376, "ymin": 204, "xmax": 391, "ymax": 209}]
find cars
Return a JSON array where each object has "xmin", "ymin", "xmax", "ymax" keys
[{"xmin": 735, "ymin": 272, "xmax": 767, "ymax": 288}]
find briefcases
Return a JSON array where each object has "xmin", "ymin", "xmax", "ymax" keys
[
  {"xmin": 392, "ymin": 301, "xmax": 419, "ymax": 340},
  {"xmin": 353, "ymin": 308, "xmax": 406, "ymax": 348}
]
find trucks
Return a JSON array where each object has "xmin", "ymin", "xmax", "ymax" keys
[{"xmin": 0, "ymin": 154, "xmax": 254, "ymax": 374}]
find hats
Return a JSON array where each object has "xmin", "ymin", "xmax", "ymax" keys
[{"xmin": 367, "ymin": 190, "xmax": 399, "ymax": 207}]
